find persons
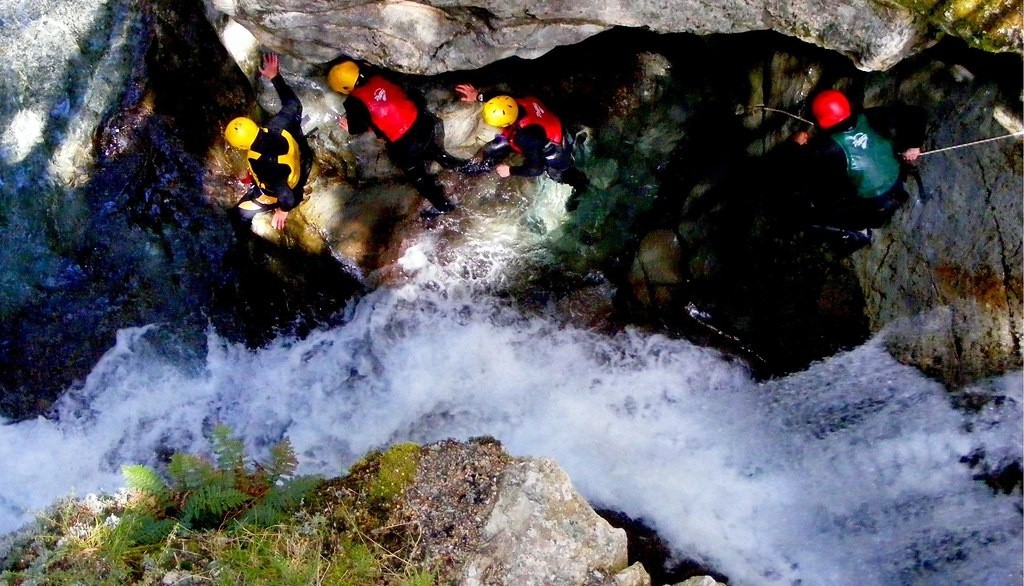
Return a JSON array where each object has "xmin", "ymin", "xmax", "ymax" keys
[
  {"xmin": 786, "ymin": 89, "xmax": 929, "ymax": 228},
  {"xmin": 224, "ymin": 52, "xmax": 314, "ymax": 242},
  {"xmin": 327, "ymin": 60, "xmax": 484, "ymax": 218},
  {"xmin": 454, "ymin": 84, "xmax": 590, "ymax": 212}
]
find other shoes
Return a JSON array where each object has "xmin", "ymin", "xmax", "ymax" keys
[{"xmin": 420, "ymin": 203, "xmax": 455, "ymax": 221}]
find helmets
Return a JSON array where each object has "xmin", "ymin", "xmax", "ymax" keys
[
  {"xmin": 328, "ymin": 60, "xmax": 361, "ymax": 96},
  {"xmin": 812, "ymin": 89, "xmax": 852, "ymax": 130},
  {"xmin": 225, "ymin": 116, "xmax": 260, "ymax": 150},
  {"xmin": 482, "ymin": 95, "xmax": 520, "ymax": 128}
]
